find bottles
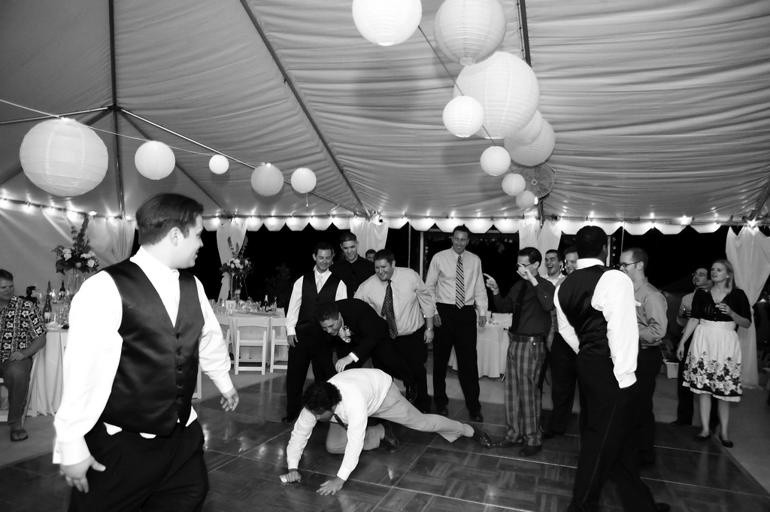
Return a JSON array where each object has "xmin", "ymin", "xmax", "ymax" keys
[{"xmin": 41, "ymin": 279, "xmax": 66, "ymax": 324}]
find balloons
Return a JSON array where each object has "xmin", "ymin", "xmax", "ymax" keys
[
  {"xmin": 351, "ymin": 0, "xmax": 422, "ymax": 46},
  {"xmin": 250, "ymin": 161, "xmax": 284, "ymax": 196},
  {"xmin": 480, "ymin": 145, "xmax": 511, "ymax": 177},
  {"xmin": 502, "ymin": 173, "xmax": 527, "ymax": 198},
  {"xmin": 516, "ymin": 188, "xmax": 536, "ymax": 212},
  {"xmin": 134, "ymin": 141, "xmax": 175, "ymax": 181},
  {"xmin": 208, "ymin": 153, "xmax": 231, "ymax": 174},
  {"xmin": 290, "ymin": 167, "xmax": 318, "ymax": 194},
  {"xmin": 440, "ymin": 96, "xmax": 485, "ymax": 139}
]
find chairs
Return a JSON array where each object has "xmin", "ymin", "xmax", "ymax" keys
[
  {"xmin": 270, "ymin": 316, "xmax": 290, "ymax": 373},
  {"xmin": 216, "ymin": 314, "xmax": 235, "ymax": 366},
  {"xmin": 232, "ymin": 315, "xmax": 270, "ymax": 377},
  {"xmin": 0, "ymin": 349, "xmax": 39, "ymax": 429}
]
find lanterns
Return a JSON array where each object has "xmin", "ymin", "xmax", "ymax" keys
[
  {"xmin": 453, "ymin": 51, "xmax": 541, "ymax": 137},
  {"xmin": 433, "ymin": 0, "xmax": 506, "ymax": 67},
  {"xmin": 504, "ymin": 108, "xmax": 558, "ymax": 167},
  {"xmin": 18, "ymin": 116, "xmax": 110, "ymax": 199}
]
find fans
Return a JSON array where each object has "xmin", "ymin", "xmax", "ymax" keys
[{"xmin": 516, "ymin": 162, "xmax": 556, "ymax": 225}]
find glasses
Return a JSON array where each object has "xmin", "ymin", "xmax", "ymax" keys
[{"xmin": 616, "ymin": 261, "xmax": 636, "ymax": 267}]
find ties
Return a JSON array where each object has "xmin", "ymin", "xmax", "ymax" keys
[
  {"xmin": 510, "ymin": 283, "xmax": 526, "ymax": 333},
  {"xmin": 382, "ymin": 280, "xmax": 398, "ymax": 339},
  {"xmin": 456, "ymin": 256, "xmax": 465, "ymax": 309}
]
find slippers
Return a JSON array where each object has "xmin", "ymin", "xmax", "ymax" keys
[{"xmin": 10, "ymin": 429, "xmax": 29, "ymax": 442}]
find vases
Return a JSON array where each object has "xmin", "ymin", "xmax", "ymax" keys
[
  {"xmin": 234, "ymin": 289, "xmax": 241, "ymax": 311},
  {"xmin": 67, "ymin": 270, "xmax": 85, "ymax": 301}
]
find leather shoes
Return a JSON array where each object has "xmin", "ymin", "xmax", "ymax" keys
[
  {"xmin": 438, "ymin": 406, "xmax": 448, "ymax": 416},
  {"xmin": 470, "ymin": 415, "xmax": 483, "ymax": 422},
  {"xmin": 464, "ymin": 422, "xmax": 492, "ymax": 448},
  {"xmin": 381, "ymin": 422, "xmax": 400, "ymax": 453},
  {"xmin": 627, "ymin": 501, "xmax": 670, "ymax": 512},
  {"xmin": 494, "ymin": 430, "xmax": 557, "ymax": 456},
  {"xmin": 669, "ymin": 421, "xmax": 734, "ymax": 447}
]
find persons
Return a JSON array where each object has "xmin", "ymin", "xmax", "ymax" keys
[
  {"xmin": 278, "ymin": 367, "xmax": 492, "ymax": 496},
  {"xmin": 554, "ymin": 225, "xmax": 679, "ymax": 512},
  {"xmin": 481, "ymin": 248, "xmax": 583, "ymax": 456},
  {"xmin": 615, "ymin": 247, "xmax": 752, "ymax": 448},
  {"xmin": 0, "ymin": 269, "xmax": 47, "ymax": 442},
  {"xmin": 279, "ymin": 232, "xmax": 432, "ymax": 415},
  {"xmin": 25, "ymin": 285, "xmax": 44, "ymax": 305},
  {"xmin": 424, "ymin": 225, "xmax": 492, "ymax": 449},
  {"xmin": 46, "ymin": 191, "xmax": 241, "ymax": 511}
]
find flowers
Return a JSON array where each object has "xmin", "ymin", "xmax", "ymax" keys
[
  {"xmin": 219, "ymin": 236, "xmax": 253, "ymax": 304},
  {"xmin": 51, "ymin": 224, "xmax": 100, "ymax": 274}
]
